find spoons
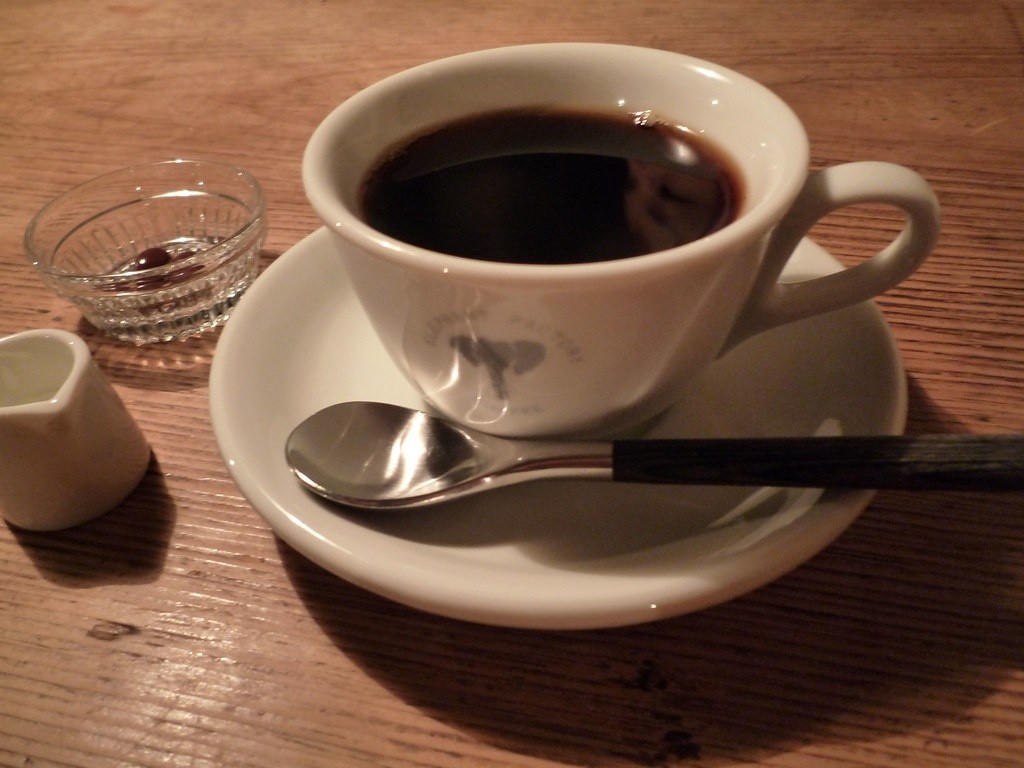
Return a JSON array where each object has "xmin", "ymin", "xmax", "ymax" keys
[{"xmin": 283, "ymin": 401, "xmax": 1024, "ymax": 510}]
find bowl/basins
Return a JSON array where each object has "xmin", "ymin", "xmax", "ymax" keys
[{"xmin": 22, "ymin": 157, "xmax": 266, "ymax": 347}]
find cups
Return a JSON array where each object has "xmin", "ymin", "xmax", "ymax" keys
[
  {"xmin": 301, "ymin": 41, "xmax": 941, "ymax": 442},
  {"xmin": 0, "ymin": 328, "xmax": 151, "ymax": 534}
]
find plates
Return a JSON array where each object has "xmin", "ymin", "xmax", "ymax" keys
[{"xmin": 205, "ymin": 224, "xmax": 918, "ymax": 629}]
